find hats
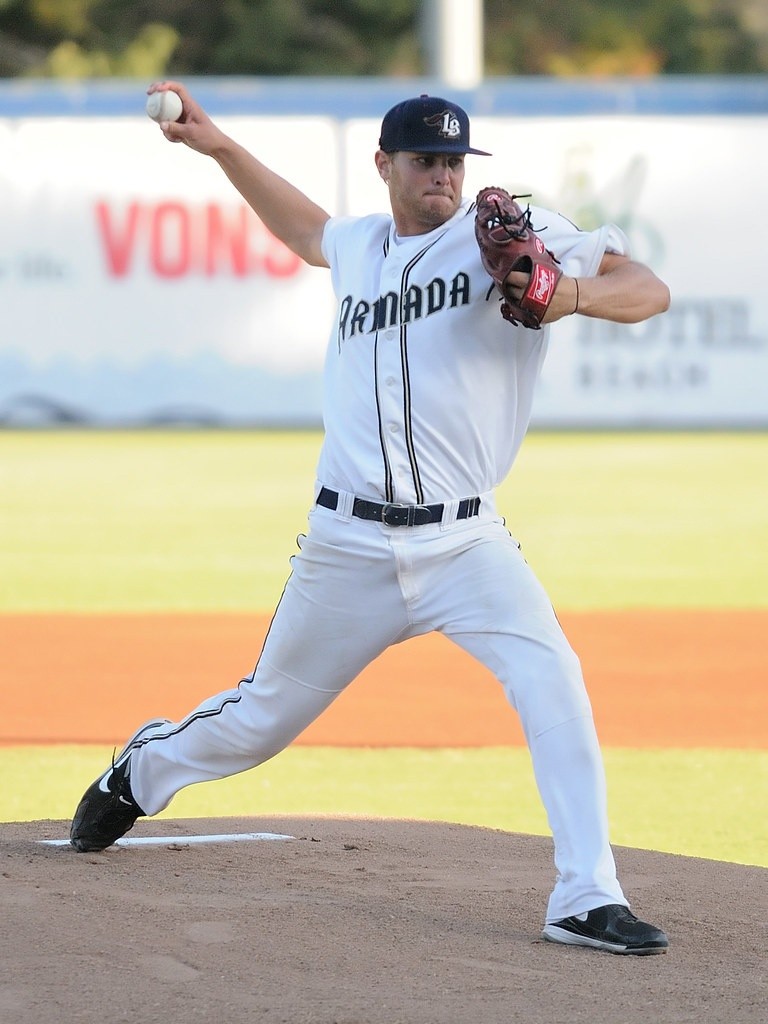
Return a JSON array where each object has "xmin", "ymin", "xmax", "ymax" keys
[{"xmin": 379, "ymin": 95, "xmax": 492, "ymax": 156}]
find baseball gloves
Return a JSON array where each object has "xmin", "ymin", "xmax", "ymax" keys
[{"xmin": 474, "ymin": 187, "xmax": 563, "ymax": 329}]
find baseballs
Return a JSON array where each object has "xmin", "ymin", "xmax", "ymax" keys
[{"xmin": 146, "ymin": 90, "xmax": 183, "ymax": 123}]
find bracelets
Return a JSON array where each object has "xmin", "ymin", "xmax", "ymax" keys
[{"xmin": 571, "ymin": 278, "xmax": 579, "ymax": 316}]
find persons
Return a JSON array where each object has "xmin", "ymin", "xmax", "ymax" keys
[{"xmin": 67, "ymin": 82, "xmax": 669, "ymax": 959}]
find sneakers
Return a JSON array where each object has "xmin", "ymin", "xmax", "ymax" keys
[
  {"xmin": 542, "ymin": 904, "xmax": 669, "ymax": 955},
  {"xmin": 69, "ymin": 718, "xmax": 174, "ymax": 853}
]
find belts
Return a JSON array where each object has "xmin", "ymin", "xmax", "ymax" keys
[{"xmin": 316, "ymin": 487, "xmax": 481, "ymax": 527}]
